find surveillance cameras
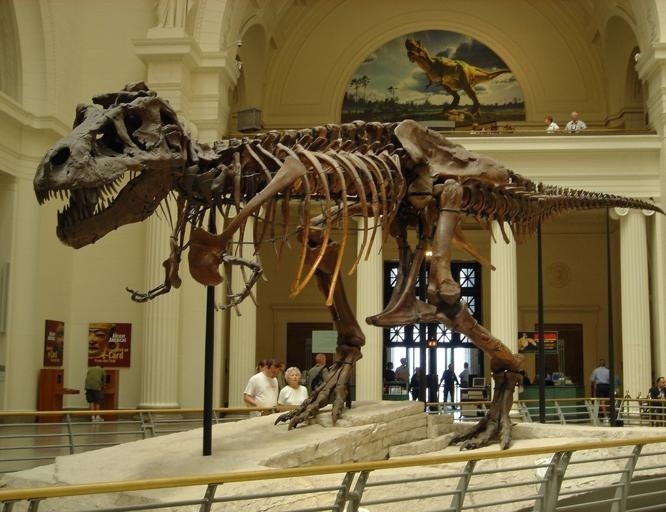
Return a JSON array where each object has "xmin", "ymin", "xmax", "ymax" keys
[{"xmin": 237, "ymin": 43, "xmax": 242, "ymax": 47}]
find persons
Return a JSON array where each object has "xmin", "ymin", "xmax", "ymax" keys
[
  {"xmin": 649, "ymin": 377, "xmax": 666, "ymax": 413},
  {"xmin": 590, "ymin": 358, "xmax": 610, "ymax": 422},
  {"xmin": 85, "ymin": 364, "xmax": 107, "ymax": 421},
  {"xmin": 470, "ymin": 120, "xmax": 513, "ymax": 135},
  {"xmin": 543, "ymin": 115, "xmax": 559, "ymax": 134},
  {"xmin": 384, "ymin": 359, "xmax": 469, "ymax": 411},
  {"xmin": 564, "ymin": 111, "xmax": 588, "ymax": 134},
  {"xmin": 244, "ymin": 353, "xmax": 329, "ymax": 418},
  {"xmin": 517, "ymin": 369, "xmax": 552, "ymax": 386},
  {"xmin": 88, "ymin": 323, "xmax": 117, "ymax": 357}
]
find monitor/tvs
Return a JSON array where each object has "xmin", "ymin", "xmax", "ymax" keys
[
  {"xmin": 473, "ymin": 378, "xmax": 486, "ymax": 387},
  {"xmin": 552, "ymin": 372, "xmax": 564, "ymax": 385}
]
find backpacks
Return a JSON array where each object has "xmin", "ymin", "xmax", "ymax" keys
[{"xmin": 311, "ymin": 365, "xmax": 328, "ymax": 391}]
